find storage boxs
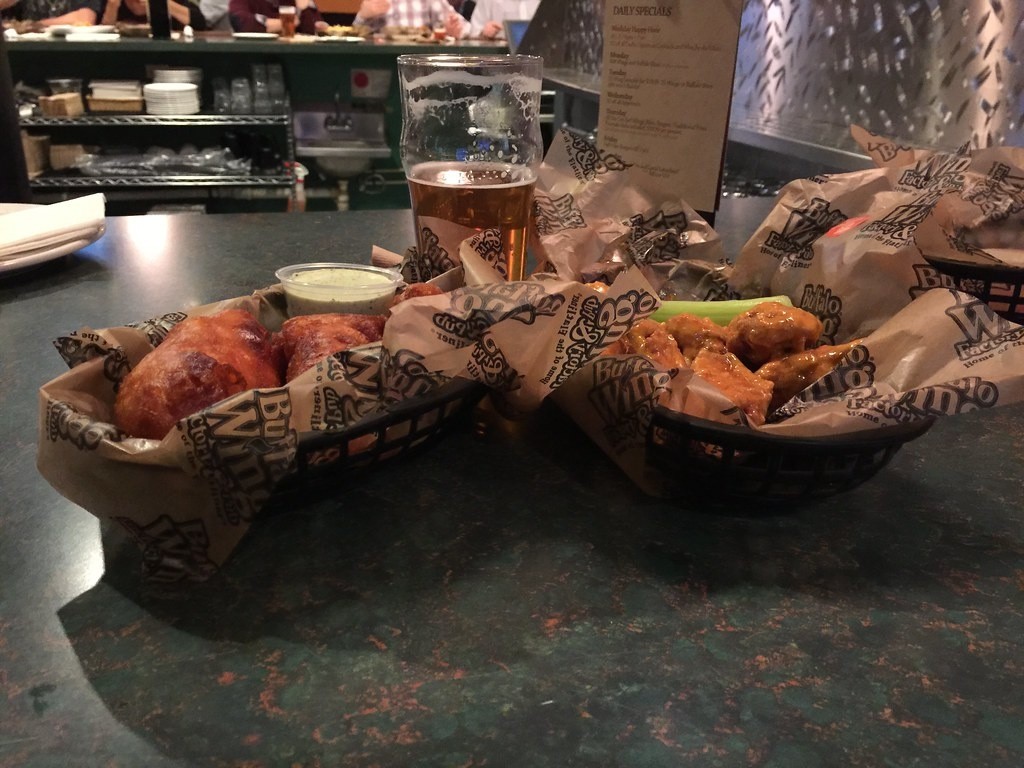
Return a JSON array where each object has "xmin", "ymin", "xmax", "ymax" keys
[{"xmin": 39, "ymin": 92, "xmax": 84, "ymax": 118}]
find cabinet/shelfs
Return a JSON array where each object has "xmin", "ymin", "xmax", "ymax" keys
[{"xmin": 20, "ymin": 116, "xmax": 299, "ymax": 212}]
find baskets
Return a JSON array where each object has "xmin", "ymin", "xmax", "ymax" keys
[
  {"xmin": 276, "ymin": 381, "xmax": 482, "ymax": 484},
  {"xmin": 655, "ymin": 401, "xmax": 935, "ymax": 500}
]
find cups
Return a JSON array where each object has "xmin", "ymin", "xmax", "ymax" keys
[
  {"xmin": 214, "ymin": 63, "xmax": 286, "ymax": 115},
  {"xmin": 278, "ymin": 5, "xmax": 297, "ymax": 38},
  {"xmin": 396, "ymin": 52, "xmax": 544, "ymax": 285},
  {"xmin": 433, "ymin": 22, "xmax": 447, "ymax": 41}
]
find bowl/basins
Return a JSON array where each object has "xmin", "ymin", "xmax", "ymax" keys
[
  {"xmin": 119, "ymin": 25, "xmax": 151, "ymax": 36},
  {"xmin": 274, "ymin": 262, "xmax": 405, "ymax": 321}
]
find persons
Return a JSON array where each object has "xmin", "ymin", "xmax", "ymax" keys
[
  {"xmin": 350, "ymin": 0, "xmax": 472, "ymax": 39},
  {"xmin": 122, "ymin": 0, "xmax": 230, "ymax": 27},
  {"xmin": 469, "ymin": 1, "xmax": 541, "ymax": 40},
  {"xmin": 0, "ymin": 0, "xmax": 104, "ymax": 36},
  {"xmin": 226, "ymin": 0, "xmax": 334, "ymax": 33}
]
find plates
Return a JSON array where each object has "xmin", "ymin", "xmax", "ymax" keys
[
  {"xmin": 144, "ymin": 64, "xmax": 204, "ymax": 116},
  {"xmin": 0, "ymin": 203, "xmax": 106, "ymax": 280},
  {"xmin": 231, "ymin": 32, "xmax": 279, "ymax": 39}
]
